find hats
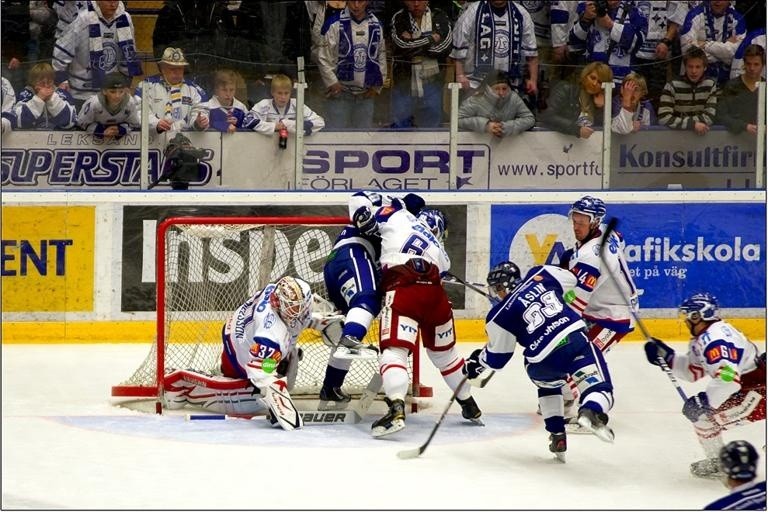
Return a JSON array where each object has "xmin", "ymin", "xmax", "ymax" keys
[
  {"xmin": 154, "ymin": 46, "xmax": 191, "ymax": 68},
  {"xmin": 99, "ymin": 71, "xmax": 130, "ymax": 90},
  {"xmin": 487, "ymin": 69, "xmax": 514, "ymax": 89}
]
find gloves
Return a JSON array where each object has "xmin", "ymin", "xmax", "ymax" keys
[
  {"xmin": 354, "ymin": 207, "xmax": 380, "ymax": 237},
  {"xmin": 681, "ymin": 390, "xmax": 711, "ymax": 424},
  {"xmin": 643, "ymin": 335, "xmax": 675, "ymax": 370},
  {"xmin": 460, "ymin": 347, "xmax": 488, "ymax": 381},
  {"xmin": 266, "ymin": 408, "xmax": 281, "ymax": 427}
]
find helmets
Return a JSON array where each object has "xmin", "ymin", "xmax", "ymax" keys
[
  {"xmin": 570, "ymin": 193, "xmax": 610, "ymax": 226},
  {"xmin": 275, "ymin": 275, "xmax": 316, "ymax": 321},
  {"xmin": 716, "ymin": 439, "xmax": 763, "ymax": 482},
  {"xmin": 675, "ymin": 291, "xmax": 723, "ymax": 324},
  {"xmin": 417, "ymin": 208, "xmax": 452, "ymax": 240},
  {"xmin": 486, "ymin": 260, "xmax": 524, "ymax": 295}
]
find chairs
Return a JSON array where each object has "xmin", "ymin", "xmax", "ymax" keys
[{"xmin": 126, "ymin": 15, "xmax": 168, "ymax": 91}]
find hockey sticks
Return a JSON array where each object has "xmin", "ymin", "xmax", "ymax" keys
[
  {"xmin": 397, "ymin": 374, "xmax": 467, "ymax": 459},
  {"xmin": 599, "ymin": 215, "xmax": 689, "ymax": 403},
  {"xmin": 188, "ymin": 373, "xmax": 382, "ymax": 425}
]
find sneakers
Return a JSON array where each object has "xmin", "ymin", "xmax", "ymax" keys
[
  {"xmin": 547, "ymin": 433, "xmax": 570, "ymax": 452},
  {"xmin": 687, "ymin": 455, "xmax": 727, "ymax": 484},
  {"xmin": 318, "ymin": 379, "xmax": 354, "ymax": 403},
  {"xmin": 577, "ymin": 404, "xmax": 617, "ymax": 442},
  {"xmin": 538, "ymin": 395, "xmax": 576, "ymax": 409},
  {"xmin": 563, "ymin": 415, "xmax": 584, "ymax": 429}
]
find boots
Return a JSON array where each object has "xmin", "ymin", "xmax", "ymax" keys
[
  {"xmin": 455, "ymin": 395, "xmax": 484, "ymax": 420},
  {"xmin": 369, "ymin": 397, "xmax": 406, "ymax": 430}
]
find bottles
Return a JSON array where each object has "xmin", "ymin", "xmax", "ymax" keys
[{"xmin": 278, "ymin": 126, "xmax": 288, "ymax": 150}]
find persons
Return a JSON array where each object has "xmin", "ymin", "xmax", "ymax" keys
[
  {"xmin": 645, "ymin": 291, "xmax": 766, "ymax": 479},
  {"xmin": 462, "ymin": 261, "xmax": 615, "ymax": 454},
  {"xmin": 349, "ymin": 187, "xmax": 482, "ymax": 437},
  {"xmin": 318, "ymin": 207, "xmax": 381, "ymax": 403},
  {"xmin": 315, "ymin": 1, "xmax": 767, "ymax": 139},
  {"xmin": 536, "ymin": 195, "xmax": 639, "ymax": 424},
  {"xmin": 2, "ymin": 1, "xmax": 324, "ymax": 139},
  {"xmin": 701, "ymin": 440, "xmax": 767, "ymax": 511},
  {"xmin": 163, "ymin": 275, "xmax": 346, "ymax": 432}
]
